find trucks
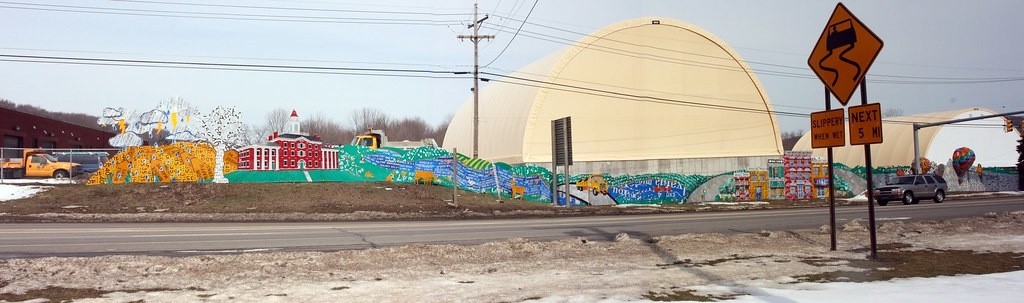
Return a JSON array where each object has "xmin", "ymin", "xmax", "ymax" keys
[
  {"xmin": 351, "ymin": 135, "xmax": 438, "ymax": 149},
  {"xmin": 0, "ymin": 148, "xmax": 82, "ymax": 180}
]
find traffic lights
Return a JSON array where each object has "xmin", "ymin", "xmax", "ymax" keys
[{"xmin": 1006, "ymin": 119, "xmax": 1013, "ymax": 131}]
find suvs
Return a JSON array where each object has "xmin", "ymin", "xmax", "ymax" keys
[{"xmin": 865, "ymin": 174, "xmax": 948, "ymax": 204}]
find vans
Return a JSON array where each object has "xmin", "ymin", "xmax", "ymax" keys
[{"xmin": 52, "ymin": 152, "xmax": 109, "ymax": 175}]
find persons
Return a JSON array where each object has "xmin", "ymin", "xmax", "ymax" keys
[
  {"xmin": 908, "ymin": 162, "xmax": 917, "ymax": 175},
  {"xmin": 975, "ymin": 164, "xmax": 984, "ymax": 182}
]
